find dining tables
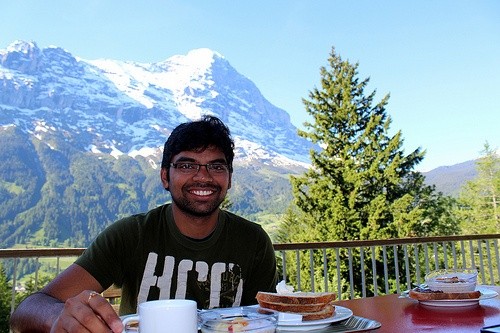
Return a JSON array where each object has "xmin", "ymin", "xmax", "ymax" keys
[{"xmin": 331, "ymin": 286, "xmax": 500, "ymax": 333}]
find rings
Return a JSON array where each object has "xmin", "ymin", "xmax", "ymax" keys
[{"xmin": 87, "ymin": 291, "xmax": 102, "ymax": 303}]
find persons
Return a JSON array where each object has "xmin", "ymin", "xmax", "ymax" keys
[{"xmin": 9, "ymin": 115, "xmax": 279, "ymax": 333}]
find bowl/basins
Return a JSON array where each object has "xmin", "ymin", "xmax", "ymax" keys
[
  {"xmin": 278, "ymin": 313, "xmax": 302, "ymax": 325},
  {"xmin": 198, "ymin": 306, "xmax": 279, "ymax": 333},
  {"xmin": 424, "ymin": 268, "xmax": 478, "ymax": 293}
]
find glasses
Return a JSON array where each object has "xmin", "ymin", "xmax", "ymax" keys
[{"xmin": 169, "ymin": 162, "xmax": 231, "ymax": 174}]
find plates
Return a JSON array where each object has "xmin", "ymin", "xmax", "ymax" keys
[
  {"xmin": 396, "ymin": 288, "xmax": 499, "ymax": 307},
  {"xmin": 247, "ymin": 304, "xmax": 354, "ymax": 331}
]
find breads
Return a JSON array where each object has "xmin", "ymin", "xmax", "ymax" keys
[
  {"xmin": 260, "ymin": 301, "xmax": 329, "ymax": 313},
  {"xmin": 256, "ymin": 291, "xmax": 336, "ymax": 304},
  {"xmin": 408, "ymin": 290, "xmax": 482, "ymax": 300},
  {"xmin": 258, "ymin": 308, "xmax": 335, "ymax": 320}
]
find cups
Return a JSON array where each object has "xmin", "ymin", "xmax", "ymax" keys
[{"xmin": 121, "ymin": 299, "xmax": 198, "ymax": 333}]
